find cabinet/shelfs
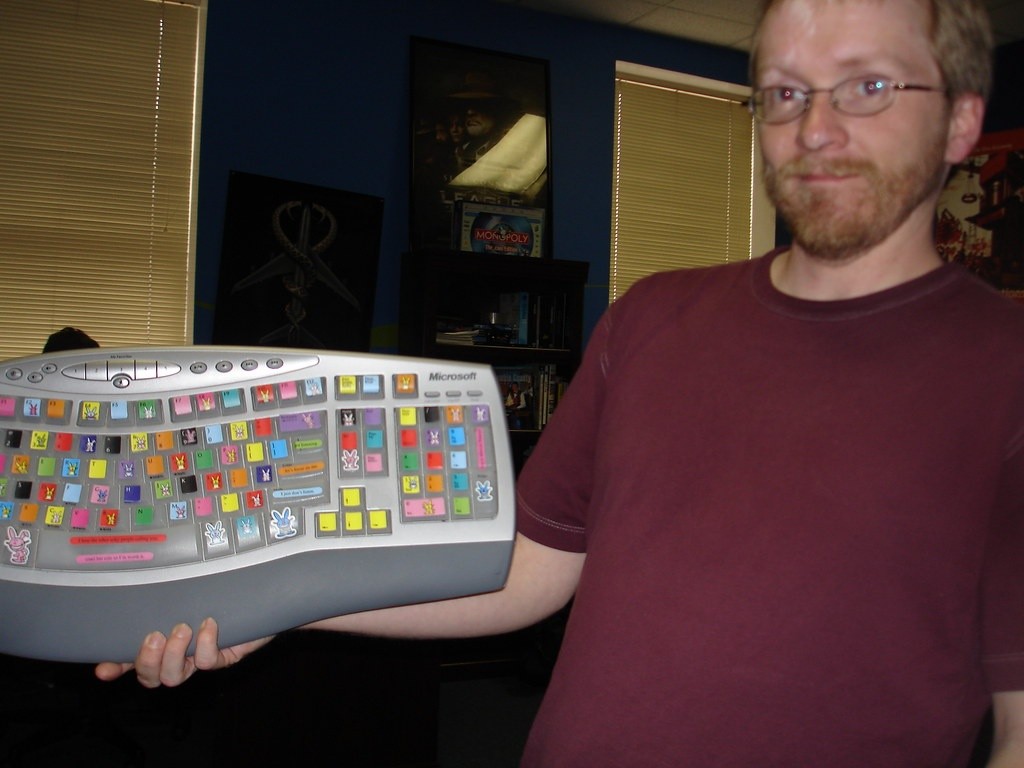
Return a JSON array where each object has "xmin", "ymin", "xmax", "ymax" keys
[{"xmin": 398, "ymin": 256, "xmax": 592, "ymax": 477}]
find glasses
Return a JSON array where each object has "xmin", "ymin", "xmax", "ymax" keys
[{"xmin": 739, "ymin": 74, "xmax": 947, "ymax": 126}]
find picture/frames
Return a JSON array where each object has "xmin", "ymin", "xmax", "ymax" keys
[{"xmin": 408, "ymin": 35, "xmax": 554, "ymax": 259}]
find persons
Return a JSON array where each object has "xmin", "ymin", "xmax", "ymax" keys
[
  {"xmin": 96, "ymin": 0, "xmax": 1023, "ymax": 768},
  {"xmin": 42, "ymin": 326, "xmax": 100, "ymax": 355}
]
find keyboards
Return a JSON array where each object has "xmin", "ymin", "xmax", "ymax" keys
[{"xmin": 0, "ymin": 346, "xmax": 518, "ymax": 663}]
